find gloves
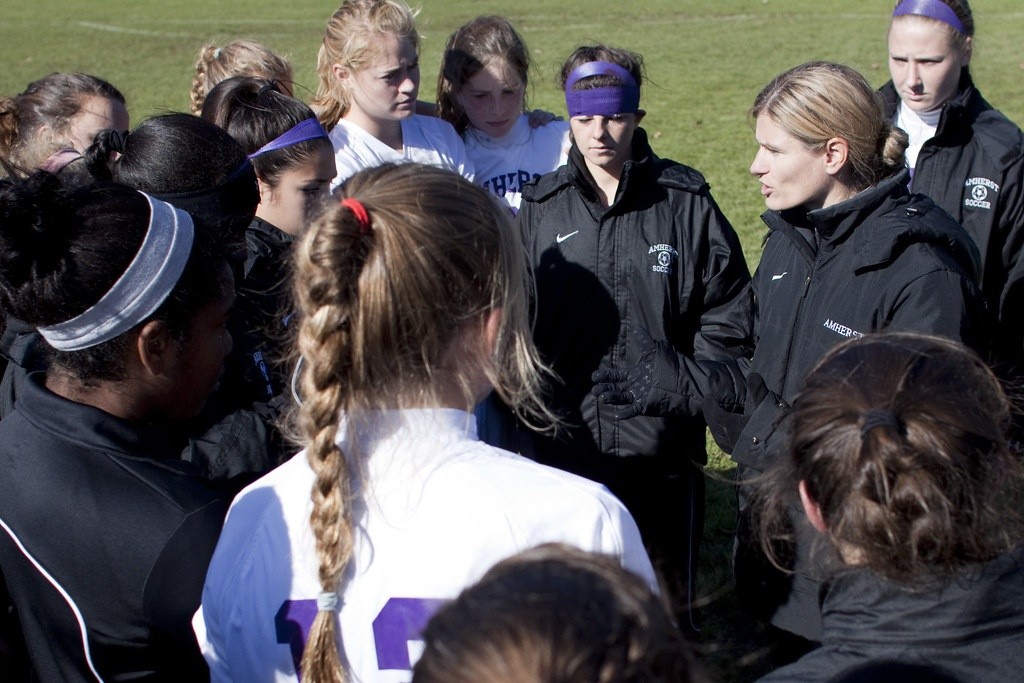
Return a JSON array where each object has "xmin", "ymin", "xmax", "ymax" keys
[
  {"xmin": 591, "ymin": 325, "xmax": 659, "ymax": 420},
  {"xmin": 700, "ymin": 371, "xmax": 770, "ymax": 455}
]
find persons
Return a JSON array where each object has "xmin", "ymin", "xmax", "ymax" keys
[
  {"xmin": 313, "ymin": 0, "xmax": 645, "ymax": 195},
  {"xmin": 191, "ymin": 69, "xmax": 343, "ymax": 394},
  {"xmin": 315, "ymin": 41, "xmax": 564, "ymax": 145},
  {"xmin": 75, "ymin": 111, "xmax": 316, "ymax": 509},
  {"xmin": 504, "ymin": 42, "xmax": 758, "ymax": 585},
  {"xmin": 0, "ymin": 169, "xmax": 232, "ymax": 683},
  {"xmin": 862, "ymin": 1, "xmax": 1024, "ymax": 418},
  {"xmin": 186, "ymin": 32, "xmax": 299, "ymax": 128},
  {"xmin": 0, "ymin": 66, "xmax": 130, "ymax": 199},
  {"xmin": 589, "ymin": 60, "xmax": 989, "ymax": 665},
  {"xmin": 746, "ymin": 323, "xmax": 1023, "ymax": 683},
  {"xmin": 405, "ymin": 539, "xmax": 711, "ymax": 683},
  {"xmin": 192, "ymin": 156, "xmax": 675, "ymax": 683}
]
